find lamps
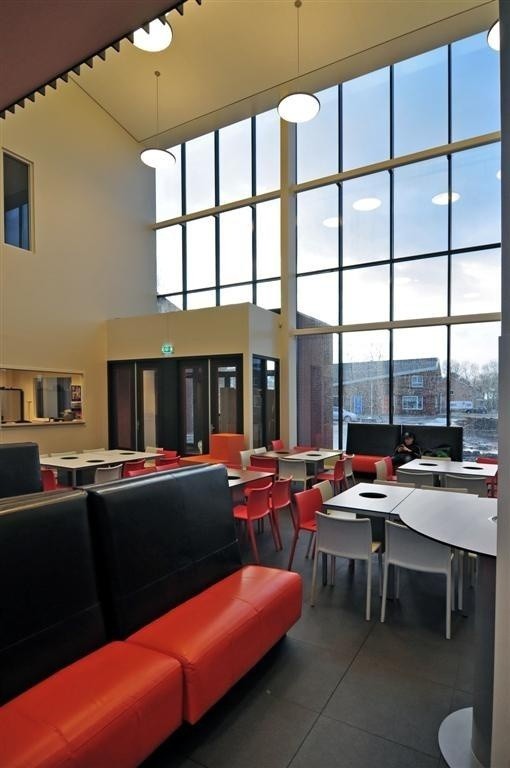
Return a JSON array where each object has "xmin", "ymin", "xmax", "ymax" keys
[
  {"xmin": 140, "ymin": 70, "xmax": 176, "ymax": 168},
  {"xmin": 488, "ymin": 19, "xmax": 502, "ymax": 52},
  {"xmin": 278, "ymin": 0, "xmax": 321, "ymax": 124},
  {"xmin": 128, "ymin": 19, "xmax": 173, "ymax": 54}
]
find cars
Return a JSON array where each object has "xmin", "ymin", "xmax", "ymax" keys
[
  {"xmin": 333, "ymin": 407, "xmax": 360, "ymax": 422},
  {"xmin": 465, "ymin": 407, "xmax": 487, "ymax": 414}
]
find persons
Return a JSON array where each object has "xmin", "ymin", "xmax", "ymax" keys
[{"xmin": 388, "ymin": 431, "xmax": 421, "ymax": 464}]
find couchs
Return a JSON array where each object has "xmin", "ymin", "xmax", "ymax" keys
[
  {"xmin": 341, "ymin": 422, "xmax": 401, "ymax": 474},
  {"xmin": 78, "ymin": 462, "xmax": 214, "ymax": 490},
  {"xmin": 179, "ymin": 433, "xmax": 245, "ymax": 469},
  {"xmin": 394, "ymin": 425, "xmax": 463, "ymax": 474},
  {"xmin": 91, "ymin": 464, "xmax": 303, "ymax": 737},
  {"xmin": 0, "ymin": 442, "xmax": 43, "ymax": 498},
  {"xmin": 0, "ymin": 488, "xmax": 75, "ymax": 505},
  {"xmin": 0, "ymin": 489, "xmax": 185, "ymax": 768}
]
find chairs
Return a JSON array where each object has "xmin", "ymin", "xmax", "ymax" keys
[
  {"xmin": 354, "ymin": 480, "xmax": 415, "ymax": 538},
  {"xmin": 243, "ymin": 466, "xmax": 277, "ymax": 502},
  {"xmin": 254, "ymin": 446, "xmax": 266, "ymax": 453},
  {"xmin": 383, "ymin": 456, "xmax": 396, "ymax": 482},
  {"xmin": 250, "ymin": 455, "xmax": 278, "ymax": 477},
  {"xmin": 145, "ymin": 446, "xmax": 156, "ymax": 467},
  {"xmin": 160, "ymin": 456, "xmax": 181, "ymax": 462},
  {"xmin": 128, "ymin": 467, "xmax": 156, "ymax": 477},
  {"xmin": 380, "ymin": 519, "xmax": 455, "ymax": 640},
  {"xmin": 375, "ymin": 460, "xmax": 387, "ymax": 481},
  {"xmin": 396, "ymin": 470, "xmax": 436, "ymax": 488},
  {"xmin": 421, "ymin": 485, "xmax": 480, "ymax": 589},
  {"xmin": 164, "ymin": 451, "xmax": 178, "ymax": 457},
  {"xmin": 233, "ymin": 481, "xmax": 280, "ymax": 564},
  {"xmin": 51, "ymin": 451, "xmax": 78, "ymax": 455},
  {"xmin": 477, "ymin": 457, "xmax": 499, "ymax": 497},
  {"xmin": 277, "ymin": 457, "xmax": 315, "ymax": 490},
  {"xmin": 316, "ymin": 458, "xmax": 348, "ymax": 495},
  {"xmin": 238, "ymin": 449, "xmax": 255, "ymax": 470},
  {"xmin": 95, "ymin": 464, "xmax": 123, "ymax": 484},
  {"xmin": 293, "ymin": 446, "xmax": 317, "ymax": 451},
  {"xmin": 123, "ymin": 459, "xmax": 146, "ymax": 478},
  {"xmin": 155, "ymin": 463, "xmax": 179, "ymax": 471},
  {"xmin": 286, "ymin": 488, "xmax": 334, "ymax": 572},
  {"xmin": 319, "ymin": 447, "xmax": 343, "ymax": 469},
  {"xmin": 260, "ymin": 475, "xmax": 299, "ymax": 548},
  {"xmin": 39, "ymin": 454, "xmax": 48, "ymax": 458},
  {"xmin": 271, "ymin": 439, "xmax": 283, "ymax": 451},
  {"xmin": 444, "ymin": 474, "xmax": 487, "ymax": 496},
  {"xmin": 82, "ymin": 448, "xmax": 106, "ymax": 452},
  {"xmin": 309, "ymin": 511, "xmax": 384, "ymax": 621},
  {"xmin": 324, "ymin": 453, "xmax": 356, "ymax": 489},
  {"xmin": 304, "ymin": 480, "xmax": 335, "ymax": 558},
  {"xmin": 42, "ymin": 470, "xmax": 68, "ymax": 491},
  {"xmin": 421, "ymin": 456, "xmax": 451, "ymax": 460}
]
